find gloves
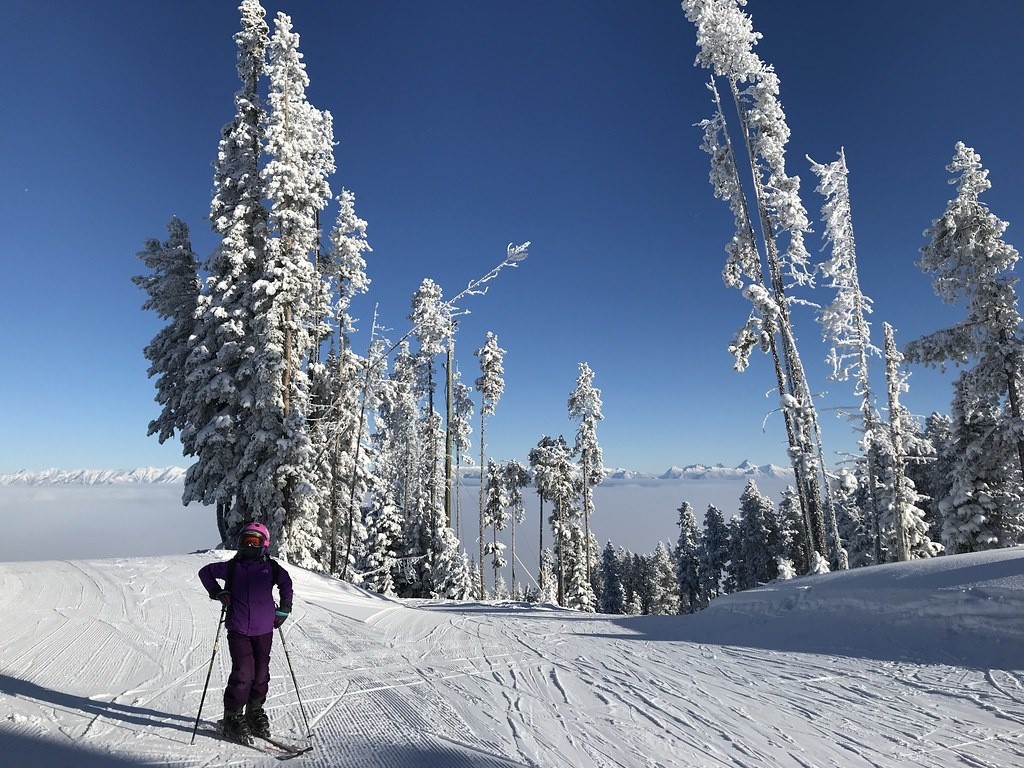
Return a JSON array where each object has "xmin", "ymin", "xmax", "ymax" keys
[
  {"xmin": 216, "ymin": 591, "xmax": 231, "ymax": 607},
  {"xmin": 273, "ymin": 610, "xmax": 290, "ymax": 629}
]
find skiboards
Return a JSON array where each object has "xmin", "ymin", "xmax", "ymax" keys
[{"xmin": 205, "ymin": 719, "xmax": 314, "ymax": 761}]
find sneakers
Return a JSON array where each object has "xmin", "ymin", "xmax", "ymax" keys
[
  {"xmin": 245, "ymin": 704, "xmax": 269, "ymax": 728},
  {"xmin": 223, "ymin": 709, "xmax": 251, "ymax": 734}
]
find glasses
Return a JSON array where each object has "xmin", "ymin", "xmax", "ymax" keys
[{"xmin": 243, "ymin": 538, "xmax": 261, "ymax": 545}]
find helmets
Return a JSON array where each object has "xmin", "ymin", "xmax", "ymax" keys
[{"xmin": 237, "ymin": 522, "xmax": 271, "ymax": 547}]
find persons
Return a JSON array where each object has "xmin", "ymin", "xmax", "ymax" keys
[{"xmin": 198, "ymin": 523, "xmax": 293, "ymax": 736}]
